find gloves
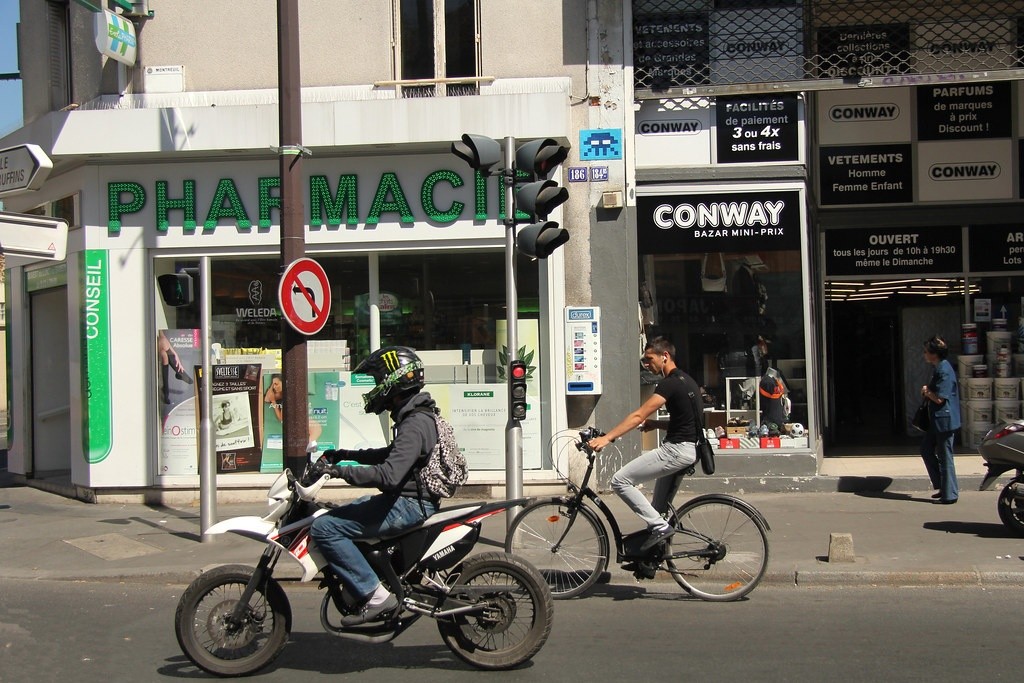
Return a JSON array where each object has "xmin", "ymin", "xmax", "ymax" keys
[
  {"xmin": 309, "ymin": 460, "xmax": 341, "ymax": 483},
  {"xmin": 314, "ymin": 447, "xmax": 348, "ymax": 466}
]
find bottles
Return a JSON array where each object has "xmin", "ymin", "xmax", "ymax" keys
[{"xmin": 996, "ymin": 345, "xmax": 1010, "ymax": 378}]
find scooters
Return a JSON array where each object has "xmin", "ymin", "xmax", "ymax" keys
[{"xmin": 977, "ymin": 417, "xmax": 1024, "ymax": 535}]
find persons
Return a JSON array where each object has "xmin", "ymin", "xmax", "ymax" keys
[
  {"xmin": 265, "ymin": 373, "xmax": 322, "ymax": 443},
  {"xmin": 588, "ymin": 335, "xmax": 704, "ymax": 579},
  {"xmin": 214, "ymin": 401, "xmax": 237, "ymax": 432},
  {"xmin": 919, "ymin": 337, "xmax": 963, "ymax": 504},
  {"xmin": 758, "ymin": 367, "xmax": 786, "ymax": 424},
  {"xmin": 317, "ymin": 346, "xmax": 443, "ymax": 626},
  {"xmin": 158, "ymin": 331, "xmax": 193, "ymax": 405}
]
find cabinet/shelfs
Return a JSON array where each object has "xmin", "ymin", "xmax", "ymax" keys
[{"xmin": 725, "ymin": 359, "xmax": 809, "ymax": 449}]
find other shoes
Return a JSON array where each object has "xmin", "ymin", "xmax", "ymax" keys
[
  {"xmin": 932, "ymin": 498, "xmax": 960, "ymax": 503},
  {"xmin": 338, "ymin": 593, "xmax": 401, "ymax": 629},
  {"xmin": 931, "ymin": 491, "xmax": 943, "ymax": 499}
]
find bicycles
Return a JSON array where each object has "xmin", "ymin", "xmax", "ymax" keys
[{"xmin": 502, "ymin": 425, "xmax": 775, "ymax": 604}]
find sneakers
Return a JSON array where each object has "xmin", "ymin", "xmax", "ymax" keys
[
  {"xmin": 620, "ymin": 562, "xmax": 656, "ymax": 579},
  {"xmin": 639, "ymin": 524, "xmax": 676, "ymax": 552}
]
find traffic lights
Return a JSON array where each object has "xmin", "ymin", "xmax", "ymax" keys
[
  {"xmin": 508, "ymin": 358, "xmax": 531, "ymax": 425},
  {"xmin": 509, "ymin": 139, "xmax": 572, "ymax": 260},
  {"xmin": 445, "ymin": 132, "xmax": 506, "ymax": 177}
]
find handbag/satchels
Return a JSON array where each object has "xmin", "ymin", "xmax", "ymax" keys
[
  {"xmin": 698, "ymin": 437, "xmax": 715, "ymax": 476},
  {"xmin": 912, "ymin": 394, "xmax": 929, "ymax": 433}
]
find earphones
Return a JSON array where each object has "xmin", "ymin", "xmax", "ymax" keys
[{"xmin": 663, "ymin": 356, "xmax": 666, "ymax": 361}]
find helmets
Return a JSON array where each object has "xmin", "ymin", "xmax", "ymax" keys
[{"xmin": 352, "ymin": 344, "xmax": 426, "ymax": 415}]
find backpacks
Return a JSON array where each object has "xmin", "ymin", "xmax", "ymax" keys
[{"xmin": 399, "ymin": 406, "xmax": 470, "ymax": 501}]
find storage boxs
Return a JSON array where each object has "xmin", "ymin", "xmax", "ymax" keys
[
  {"xmin": 761, "ymin": 437, "xmax": 780, "ymax": 448},
  {"xmin": 780, "ymin": 435, "xmax": 808, "ymax": 449},
  {"xmin": 720, "ymin": 438, "xmax": 740, "ymax": 450},
  {"xmin": 708, "ymin": 439, "xmax": 718, "ymax": 450},
  {"xmin": 740, "ymin": 435, "xmax": 760, "ymax": 449},
  {"xmin": 980, "ymin": 425, "xmax": 1024, "ymax": 465}
]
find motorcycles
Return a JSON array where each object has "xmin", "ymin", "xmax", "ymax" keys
[{"xmin": 170, "ymin": 439, "xmax": 557, "ymax": 676}]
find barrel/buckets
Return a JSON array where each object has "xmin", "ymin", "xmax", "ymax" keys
[
  {"xmin": 992, "ymin": 319, "xmax": 1008, "ymax": 332},
  {"xmin": 1014, "ymin": 354, "xmax": 1024, "ymax": 377},
  {"xmin": 972, "ymin": 365, "xmax": 988, "ymax": 377},
  {"xmin": 961, "ymin": 323, "xmax": 978, "ymax": 355},
  {"xmin": 986, "ymin": 354, "xmax": 1013, "ymax": 377},
  {"xmin": 958, "ymin": 378, "xmax": 1024, "ymax": 452},
  {"xmin": 957, "ymin": 354, "xmax": 983, "ymax": 377},
  {"xmin": 985, "ymin": 331, "xmax": 1011, "ymax": 354}
]
